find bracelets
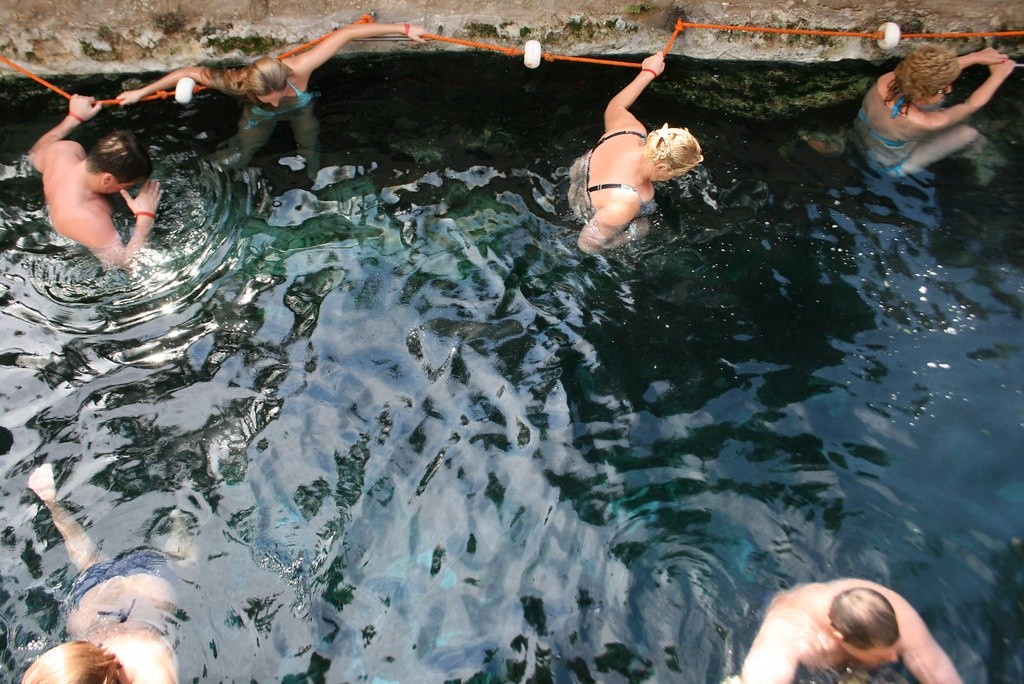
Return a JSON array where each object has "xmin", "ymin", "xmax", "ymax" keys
[
  {"xmin": 68, "ymin": 111, "xmax": 85, "ymax": 125},
  {"xmin": 136, "ymin": 211, "xmax": 158, "ymax": 220},
  {"xmin": 404, "ymin": 24, "xmax": 410, "ymax": 36},
  {"xmin": 641, "ymin": 68, "xmax": 658, "ymax": 79}
]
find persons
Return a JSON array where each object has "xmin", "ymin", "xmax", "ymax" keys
[
  {"xmin": 851, "ymin": 45, "xmax": 1014, "ymax": 176},
  {"xmin": 568, "ymin": 50, "xmax": 704, "ymax": 256},
  {"xmin": 740, "ymin": 578, "xmax": 963, "ymax": 684},
  {"xmin": 26, "ymin": 94, "xmax": 164, "ymax": 273},
  {"xmin": 19, "ymin": 461, "xmax": 199, "ymax": 684},
  {"xmin": 116, "ymin": 21, "xmax": 426, "ymax": 175}
]
